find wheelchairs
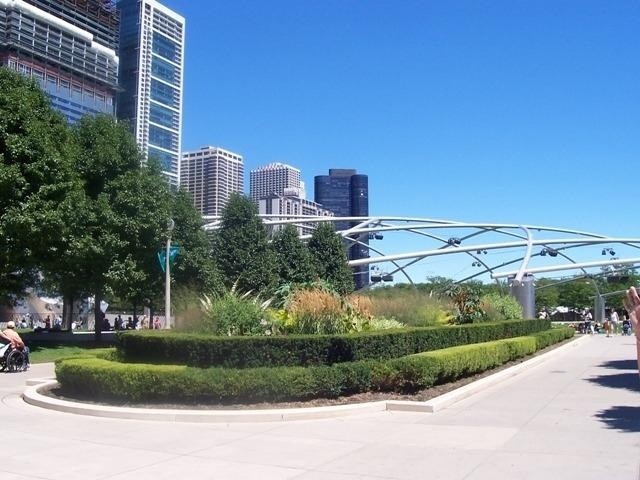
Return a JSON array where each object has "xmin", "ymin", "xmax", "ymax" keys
[{"xmin": 0, "ymin": 330, "xmax": 30, "ymax": 372}]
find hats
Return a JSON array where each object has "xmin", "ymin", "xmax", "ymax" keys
[{"xmin": 7, "ymin": 321, "xmax": 15, "ymax": 328}]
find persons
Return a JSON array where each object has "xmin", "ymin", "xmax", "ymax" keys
[
  {"xmin": 17, "ymin": 313, "xmax": 62, "ymax": 328},
  {"xmin": 103, "ymin": 314, "xmax": 162, "ymax": 330},
  {"xmin": 622, "ymin": 286, "xmax": 640, "ymax": 374},
  {"xmin": 2, "ymin": 321, "xmax": 29, "ymax": 367},
  {"xmin": 0, "ymin": 330, "xmax": 13, "ymax": 343},
  {"xmin": 583, "ymin": 307, "xmax": 632, "ymax": 337}
]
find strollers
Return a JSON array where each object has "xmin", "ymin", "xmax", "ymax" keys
[{"xmin": 621, "ymin": 320, "xmax": 632, "ymax": 335}]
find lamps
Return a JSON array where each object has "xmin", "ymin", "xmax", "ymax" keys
[{"xmin": 365, "ymin": 232, "xmax": 617, "ymax": 284}]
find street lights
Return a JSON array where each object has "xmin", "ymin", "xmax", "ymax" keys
[{"xmin": 161, "ymin": 217, "xmax": 177, "ymax": 331}]
